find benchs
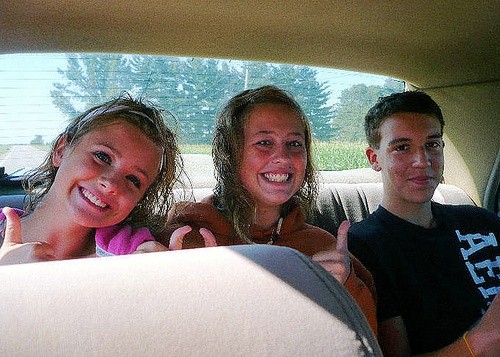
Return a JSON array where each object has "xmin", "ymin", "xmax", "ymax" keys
[{"xmin": 0, "ymin": 153, "xmax": 479, "ymax": 237}]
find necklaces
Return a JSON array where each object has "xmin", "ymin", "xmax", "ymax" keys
[{"xmin": 233, "ymin": 206, "xmax": 284, "ymax": 246}]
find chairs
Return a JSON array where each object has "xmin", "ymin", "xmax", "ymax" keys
[{"xmin": 1, "ymin": 243, "xmax": 384, "ymax": 357}]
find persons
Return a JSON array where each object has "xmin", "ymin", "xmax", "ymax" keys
[
  {"xmin": 340, "ymin": 91, "xmax": 500, "ymax": 357},
  {"xmin": 152, "ymin": 84, "xmax": 378, "ymax": 349},
  {"xmin": 0, "ymin": 90, "xmax": 193, "ymax": 265}
]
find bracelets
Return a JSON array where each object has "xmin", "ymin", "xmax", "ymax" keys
[{"xmin": 461, "ymin": 331, "xmax": 477, "ymax": 357}]
